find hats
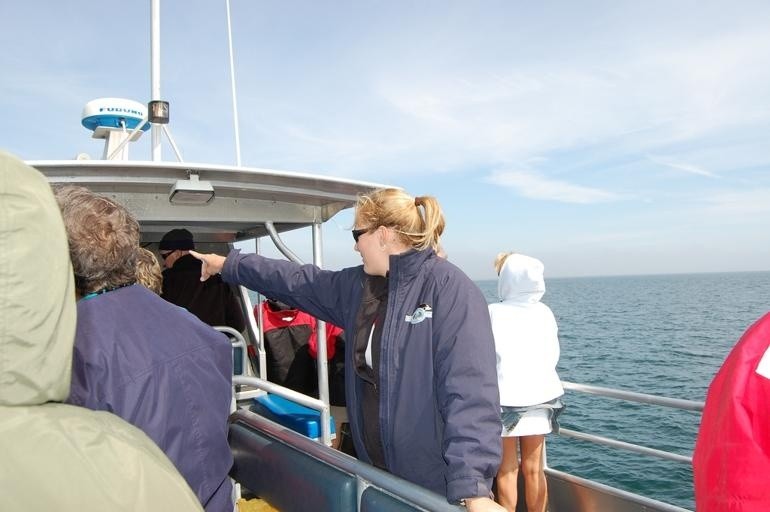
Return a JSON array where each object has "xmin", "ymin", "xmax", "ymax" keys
[{"xmin": 159, "ymin": 228, "xmax": 194, "ymax": 250}]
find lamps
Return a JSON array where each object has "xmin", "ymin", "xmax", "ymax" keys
[
  {"xmin": 168, "ymin": 171, "xmax": 215, "ymax": 207},
  {"xmin": 147, "ymin": 100, "xmax": 170, "ymax": 124}
]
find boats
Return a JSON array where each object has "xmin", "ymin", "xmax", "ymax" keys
[{"xmin": 0, "ymin": 0, "xmax": 716, "ymax": 511}]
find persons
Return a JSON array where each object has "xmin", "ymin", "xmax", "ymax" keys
[
  {"xmin": 132, "ymin": 226, "xmax": 348, "ymax": 407},
  {"xmin": 488, "ymin": 253, "xmax": 565, "ymax": 512},
  {"xmin": 53, "ymin": 185, "xmax": 234, "ymax": 511},
  {"xmin": 0, "ymin": 154, "xmax": 203, "ymax": 510},
  {"xmin": 190, "ymin": 188, "xmax": 509, "ymax": 511}
]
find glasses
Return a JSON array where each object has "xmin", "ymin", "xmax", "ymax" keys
[
  {"xmin": 352, "ymin": 228, "xmax": 375, "ymax": 242},
  {"xmin": 161, "ymin": 249, "xmax": 176, "ymax": 260}
]
